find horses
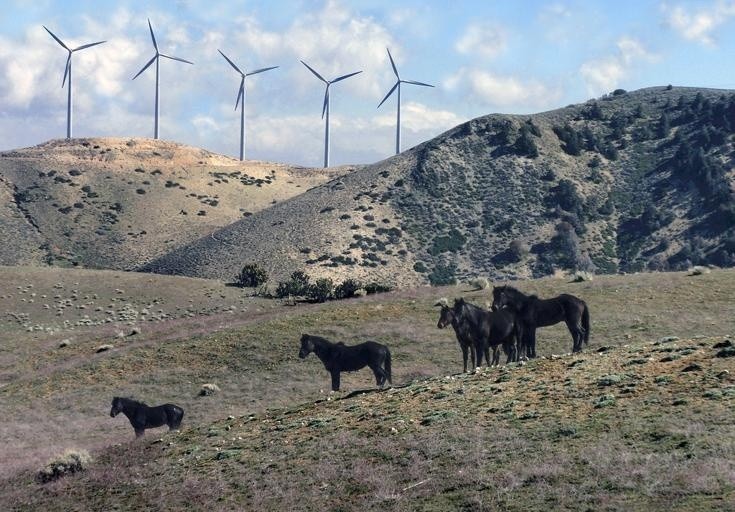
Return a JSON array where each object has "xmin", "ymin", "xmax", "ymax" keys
[
  {"xmin": 489, "ymin": 284, "xmax": 591, "ymax": 358},
  {"xmin": 435, "ymin": 296, "xmax": 516, "ymax": 373},
  {"xmin": 108, "ymin": 395, "xmax": 185, "ymax": 439},
  {"xmin": 297, "ymin": 333, "xmax": 392, "ymax": 391}
]
